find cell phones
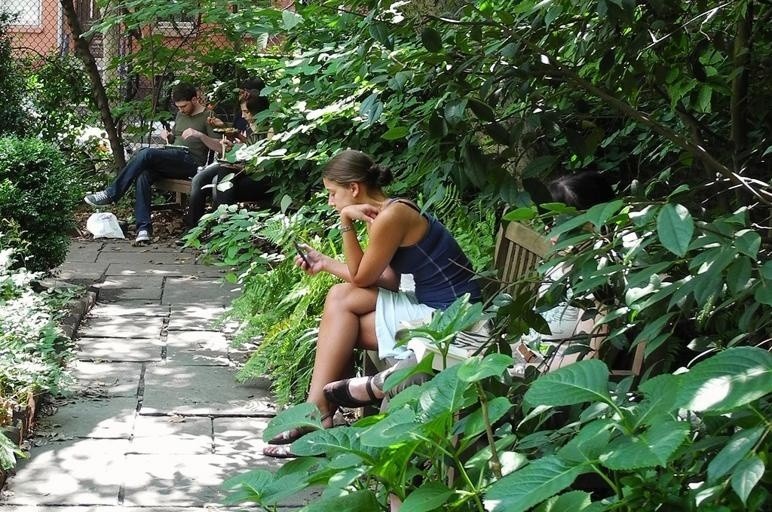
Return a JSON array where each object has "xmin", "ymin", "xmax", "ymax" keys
[{"xmin": 292, "ymin": 239, "xmax": 310, "ymax": 270}]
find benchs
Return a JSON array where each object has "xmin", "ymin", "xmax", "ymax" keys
[
  {"xmin": 150, "ymin": 177, "xmax": 193, "ymax": 211},
  {"xmin": 361, "ymin": 204, "xmax": 664, "ymax": 420}
]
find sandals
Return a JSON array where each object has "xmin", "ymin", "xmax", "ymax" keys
[{"xmin": 263, "ymin": 411, "xmax": 334, "ymax": 458}]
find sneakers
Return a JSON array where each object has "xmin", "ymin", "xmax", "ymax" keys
[
  {"xmin": 135, "ymin": 229, "xmax": 152, "ymax": 243},
  {"xmin": 84, "ymin": 189, "xmax": 113, "ymax": 207}
]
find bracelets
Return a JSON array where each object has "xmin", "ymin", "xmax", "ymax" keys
[{"xmin": 337, "ymin": 223, "xmax": 354, "ymax": 235}]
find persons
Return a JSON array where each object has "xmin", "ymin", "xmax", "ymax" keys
[
  {"xmin": 323, "ymin": 167, "xmax": 616, "ymax": 511},
  {"xmin": 200, "ymin": 89, "xmax": 312, "ymax": 264},
  {"xmin": 262, "ymin": 150, "xmax": 487, "ymax": 459},
  {"xmin": 174, "ymin": 75, "xmax": 266, "ymax": 246},
  {"xmin": 83, "ymin": 83, "xmax": 228, "ymax": 245}
]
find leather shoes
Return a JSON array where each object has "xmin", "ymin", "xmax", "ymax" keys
[{"xmin": 322, "ymin": 376, "xmax": 383, "ymax": 408}]
